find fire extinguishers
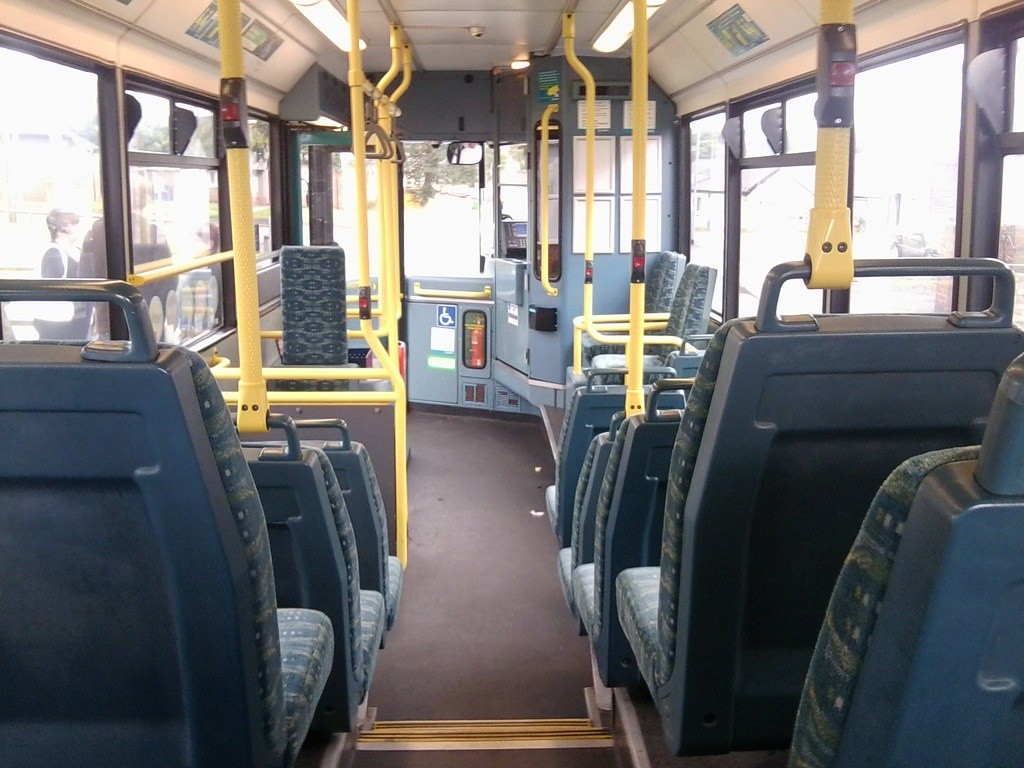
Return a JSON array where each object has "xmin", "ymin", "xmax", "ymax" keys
[{"xmin": 468, "ymin": 313, "xmax": 486, "ymax": 368}]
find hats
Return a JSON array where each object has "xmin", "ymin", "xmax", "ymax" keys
[{"xmin": 197, "ymin": 222, "xmax": 220, "ymax": 247}]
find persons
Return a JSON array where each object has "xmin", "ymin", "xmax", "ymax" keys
[
  {"xmin": 33, "ymin": 209, "xmax": 93, "ymax": 340},
  {"xmin": 76, "ymin": 174, "xmax": 179, "ymax": 343},
  {"xmin": 176, "ymin": 222, "xmax": 223, "ymax": 341}
]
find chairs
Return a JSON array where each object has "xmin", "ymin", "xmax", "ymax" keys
[
  {"xmin": 614, "ymin": 257, "xmax": 1024, "ymax": 754},
  {"xmin": 543, "ymin": 366, "xmax": 677, "ymax": 550},
  {"xmin": 591, "ymin": 264, "xmax": 717, "ymax": 387},
  {"xmin": 572, "ymin": 376, "xmax": 697, "ymax": 690},
  {"xmin": 786, "ymin": 350, "xmax": 1024, "ymax": 768},
  {"xmin": 291, "ymin": 417, "xmax": 406, "ymax": 648},
  {"xmin": 577, "ymin": 250, "xmax": 687, "ymax": 365},
  {"xmin": 269, "ymin": 244, "xmax": 361, "ymax": 392},
  {"xmin": 557, "ymin": 411, "xmax": 628, "ymax": 637},
  {"xmin": 0, "ymin": 277, "xmax": 338, "ymax": 768},
  {"xmin": 228, "ymin": 411, "xmax": 389, "ymax": 727}
]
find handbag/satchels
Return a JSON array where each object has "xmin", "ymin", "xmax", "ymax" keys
[{"xmin": 30, "ymin": 242, "xmax": 75, "ymax": 323}]
